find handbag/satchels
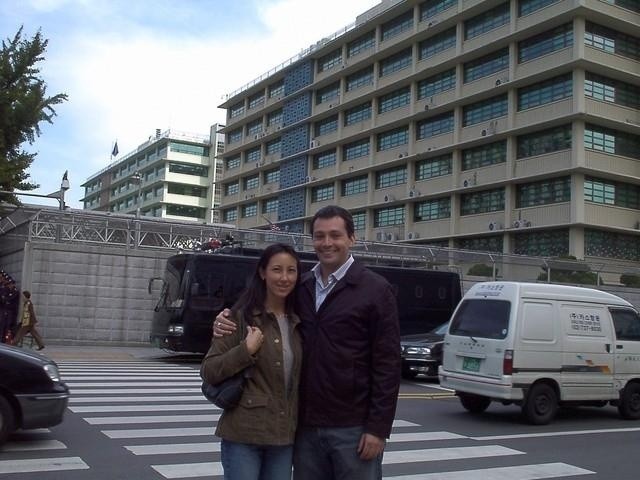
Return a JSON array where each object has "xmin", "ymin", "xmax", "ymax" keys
[{"xmin": 201, "ymin": 305, "xmax": 257, "ymax": 410}]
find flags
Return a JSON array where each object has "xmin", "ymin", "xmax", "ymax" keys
[{"xmin": 111, "ymin": 143, "xmax": 119, "ymax": 156}]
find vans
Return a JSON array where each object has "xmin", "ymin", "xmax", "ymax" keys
[{"xmin": 438, "ymin": 281, "xmax": 640, "ymax": 425}]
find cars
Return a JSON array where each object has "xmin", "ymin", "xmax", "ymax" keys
[
  {"xmin": 400, "ymin": 322, "xmax": 449, "ymax": 381},
  {"xmin": 0, "ymin": 342, "xmax": 70, "ymax": 443}
]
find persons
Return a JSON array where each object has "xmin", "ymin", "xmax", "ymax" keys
[
  {"xmin": 13, "ymin": 290, "xmax": 45, "ymax": 351},
  {"xmin": 0, "ymin": 271, "xmax": 20, "ymax": 343},
  {"xmin": 213, "ymin": 204, "xmax": 402, "ymax": 479},
  {"xmin": 198, "ymin": 243, "xmax": 304, "ymax": 479}
]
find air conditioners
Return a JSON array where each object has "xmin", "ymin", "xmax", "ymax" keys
[
  {"xmin": 460, "ymin": 178, "xmax": 472, "ymax": 187},
  {"xmin": 425, "ymin": 146, "xmax": 436, "ymax": 151},
  {"xmin": 486, "ymin": 221, "xmax": 500, "ymax": 231},
  {"xmin": 424, "ymin": 105, "xmax": 435, "ymax": 110},
  {"xmin": 479, "ymin": 129, "xmax": 490, "ymax": 136},
  {"xmin": 494, "ymin": 77, "xmax": 507, "ymax": 85},
  {"xmin": 513, "ymin": 220, "xmax": 524, "ymax": 228},
  {"xmin": 244, "ymin": 102, "xmax": 420, "ymax": 242}
]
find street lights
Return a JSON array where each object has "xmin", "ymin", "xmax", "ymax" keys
[{"xmin": 128, "ymin": 171, "xmax": 144, "ymax": 247}]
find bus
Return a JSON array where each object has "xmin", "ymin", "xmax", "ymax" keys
[{"xmin": 148, "ymin": 252, "xmax": 461, "ymax": 354}]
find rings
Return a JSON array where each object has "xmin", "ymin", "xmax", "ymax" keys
[{"xmin": 218, "ymin": 322, "xmax": 221, "ymax": 327}]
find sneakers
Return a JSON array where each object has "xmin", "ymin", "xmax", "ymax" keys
[{"xmin": 36, "ymin": 345, "xmax": 45, "ymax": 351}]
